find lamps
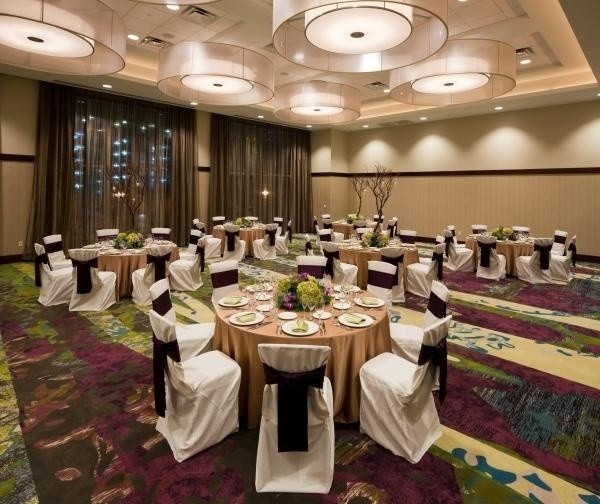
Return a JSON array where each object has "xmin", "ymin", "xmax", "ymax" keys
[
  {"xmin": 127, "ymin": 0, "xmax": 223, "ymax": 6},
  {"xmin": 0, "ymin": 0, "xmax": 127, "ymax": 77},
  {"xmin": 269, "ymin": 76, "xmax": 363, "ymax": 129},
  {"xmin": 155, "ymin": 18, "xmax": 279, "ymax": 106},
  {"xmin": 271, "ymin": 0, "xmax": 450, "ymax": 74},
  {"xmin": 388, "ymin": 0, "xmax": 518, "ymax": 108}
]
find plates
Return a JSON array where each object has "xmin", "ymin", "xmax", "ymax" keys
[
  {"xmin": 218, "ymin": 283, "xmax": 385, "ymax": 336},
  {"xmin": 341, "ymin": 239, "xmax": 416, "ymax": 252},
  {"xmin": 468, "ymin": 233, "xmax": 534, "ymax": 244},
  {"xmin": 83, "ymin": 239, "xmax": 172, "ymax": 254}
]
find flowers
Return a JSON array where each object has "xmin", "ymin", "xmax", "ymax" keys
[{"xmin": 272, "ymin": 271, "xmax": 333, "ymax": 312}]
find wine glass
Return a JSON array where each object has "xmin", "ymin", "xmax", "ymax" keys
[{"xmin": 247, "ymin": 272, "xmax": 357, "ymax": 323}]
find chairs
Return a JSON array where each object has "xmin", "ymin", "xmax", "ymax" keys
[
  {"xmin": 148, "ymin": 278, "xmax": 215, "ymax": 418},
  {"xmin": 34, "ymin": 218, "xmax": 208, "ymax": 313},
  {"xmin": 208, "ymin": 215, "xmax": 292, "ymax": 264},
  {"xmin": 148, "ymin": 308, "xmax": 243, "ymax": 465},
  {"xmin": 254, "ymin": 343, "xmax": 337, "ymax": 495},
  {"xmin": 357, "ymin": 314, "xmax": 453, "ymax": 465},
  {"xmin": 296, "ymin": 213, "xmax": 577, "ymax": 303},
  {"xmin": 207, "ymin": 260, "xmax": 241, "ymax": 310},
  {"xmin": 388, "ymin": 279, "xmax": 450, "ymax": 401}
]
